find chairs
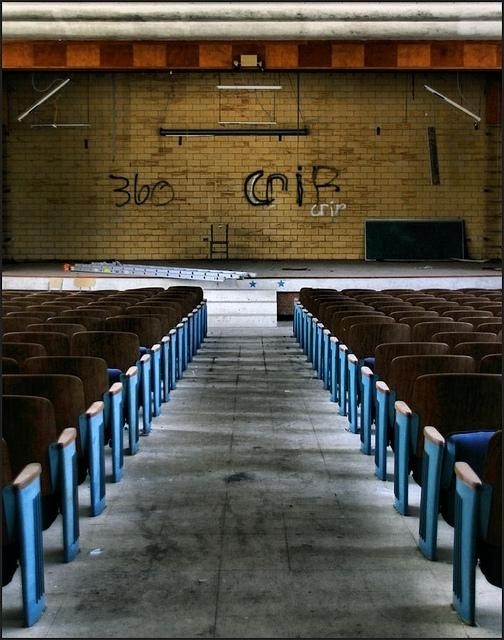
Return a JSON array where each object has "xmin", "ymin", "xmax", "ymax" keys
[
  {"xmin": 292, "ymin": 286, "xmax": 504, "ymax": 627},
  {"xmin": 0, "ymin": 285, "xmax": 209, "ymax": 630}
]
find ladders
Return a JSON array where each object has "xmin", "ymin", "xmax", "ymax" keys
[{"xmin": 64, "ymin": 261, "xmax": 255, "ymax": 283}]
